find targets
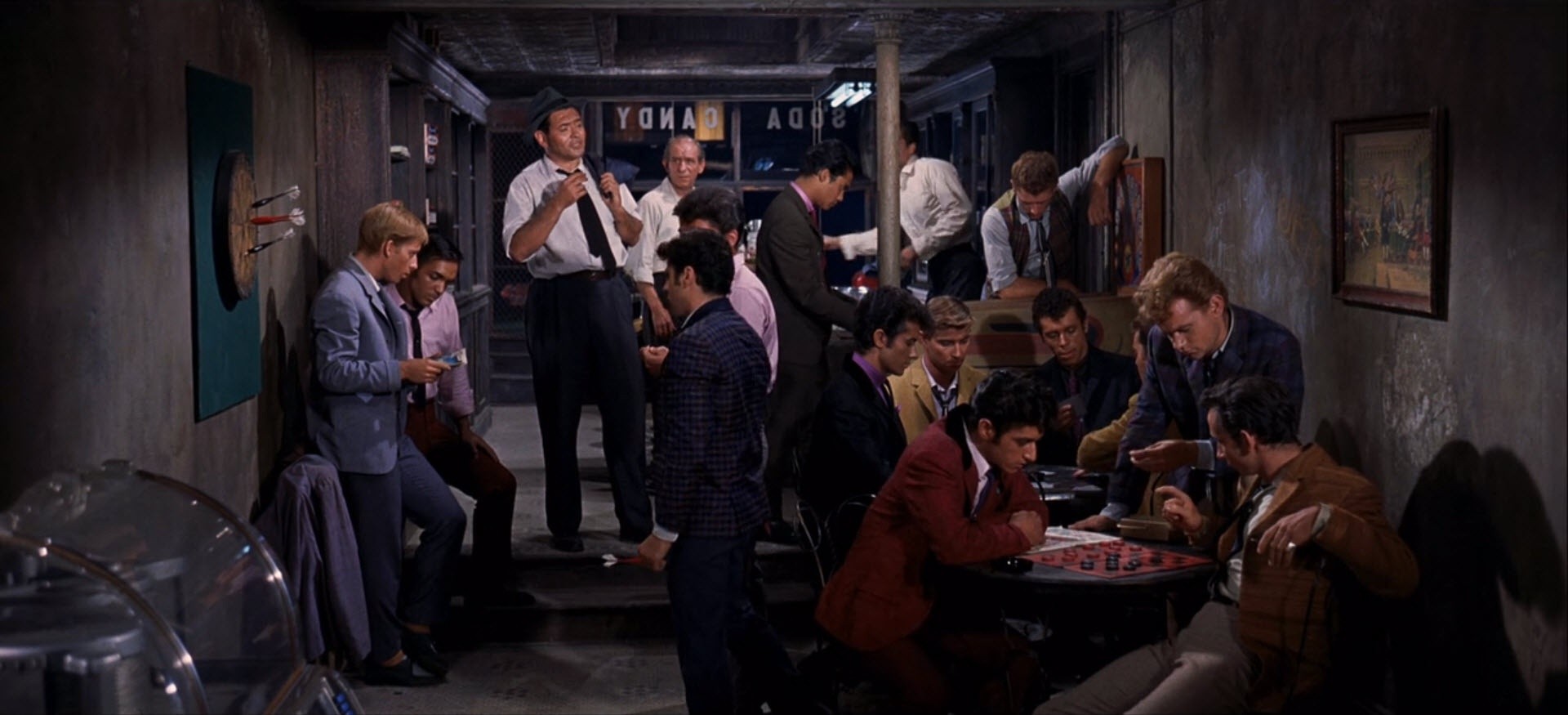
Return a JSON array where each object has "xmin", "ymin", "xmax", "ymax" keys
[{"xmin": 220, "ymin": 153, "xmax": 261, "ymax": 296}]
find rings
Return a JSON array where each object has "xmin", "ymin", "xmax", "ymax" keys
[{"xmin": 1286, "ymin": 541, "xmax": 1297, "ymax": 552}]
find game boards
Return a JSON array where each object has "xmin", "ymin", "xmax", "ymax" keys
[{"xmin": 1015, "ymin": 539, "xmax": 1211, "ymax": 579}]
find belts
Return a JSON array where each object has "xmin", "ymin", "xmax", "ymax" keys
[{"xmin": 554, "ymin": 266, "xmax": 627, "ymax": 283}]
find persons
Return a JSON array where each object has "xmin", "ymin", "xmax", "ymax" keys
[
  {"xmin": 627, "ymin": 135, "xmax": 707, "ymax": 397},
  {"xmin": 377, "ymin": 235, "xmax": 535, "ymax": 610},
  {"xmin": 1030, "ymin": 374, "xmax": 1421, "ymax": 714},
  {"xmin": 755, "ymin": 138, "xmax": 859, "ymax": 419},
  {"xmin": 807, "ymin": 284, "xmax": 934, "ymax": 595},
  {"xmin": 815, "ymin": 371, "xmax": 1058, "ymax": 715},
  {"xmin": 1026, "ymin": 252, "xmax": 1305, "ymax": 531},
  {"xmin": 306, "ymin": 197, "xmax": 467, "ymax": 687},
  {"xmin": 884, "ymin": 293, "xmax": 987, "ymax": 445},
  {"xmin": 980, "ymin": 134, "xmax": 1131, "ymax": 302},
  {"xmin": 640, "ymin": 184, "xmax": 778, "ymax": 477},
  {"xmin": 638, "ymin": 226, "xmax": 783, "ymax": 715},
  {"xmin": 822, "ymin": 121, "xmax": 988, "ymax": 303},
  {"xmin": 500, "ymin": 85, "xmax": 654, "ymax": 560}
]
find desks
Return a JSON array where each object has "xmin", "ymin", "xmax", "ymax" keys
[{"xmin": 959, "ymin": 465, "xmax": 1223, "ymax": 642}]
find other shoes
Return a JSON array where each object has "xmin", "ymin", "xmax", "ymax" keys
[
  {"xmin": 397, "ymin": 625, "xmax": 450, "ymax": 675},
  {"xmin": 472, "ymin": 587, "xmax": 535, "ymax": 608},
  {"xmin": 553, "ymin": 528, "xmax": 584, "ymax": 551},
  {"xmin": 380, "ymin": 656, "xmax": 419, "ymax": 685}
]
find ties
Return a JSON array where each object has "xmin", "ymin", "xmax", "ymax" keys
[
  {"xmin": 558, "ymin": 169, "xmax": 619, "ymax": 276},
  {"xmin": 809, "ymin": 209, "xmax": 825, "ymax": 266},
  {"xmin": 932, "ymin": 385, "xmax": 956, "ymax": 416},
  {"xmin": 401, "ymin": 303, "xmax": 426, "ymax": 411},
  {"xmin": 1030, "ymin": 216, "xmax": 1046, "ymax": 254}
]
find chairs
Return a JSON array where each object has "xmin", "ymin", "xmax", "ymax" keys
[
  {"xmin": 797, "ymin": 498, "xmax": 924, "ymax": 715},
  {"xmin": 1297, "ymin": 440, "xmax": 1490, "ymax": 715}
]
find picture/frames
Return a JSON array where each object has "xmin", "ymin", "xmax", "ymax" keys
[{"xmin": 1330, "ymin": 106, "xmax": 1448, "ymax": 314}]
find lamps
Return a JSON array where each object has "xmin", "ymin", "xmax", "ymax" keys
[{"xmin": 813, "ymin": 67, "xmax": 877, "ymax": 107}]
